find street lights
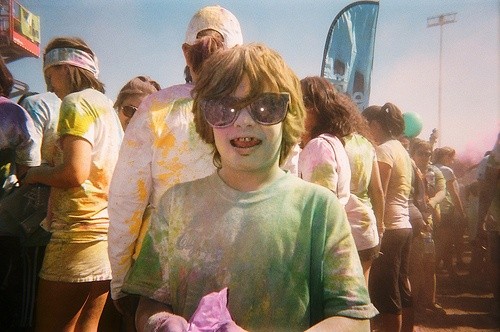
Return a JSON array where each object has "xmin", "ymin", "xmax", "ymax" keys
[{"xmin": 427, "ymin": 11, "xmax": 458, "ymax": 150}]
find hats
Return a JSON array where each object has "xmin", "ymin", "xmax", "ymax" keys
[{"xmin": 184, "ymin": 4, "xmax": 244, "ymax": 52}]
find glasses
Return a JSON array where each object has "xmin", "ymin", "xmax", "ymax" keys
[
  {"xmin": 416, "ymin": 150, "xmax": 431, "ymax": 158},
  {"xmin": 199, "ymin": 92, "xmax": 291, "ymax": 129},
  {"xmin": 120, "ymin": 104, "xmax": 138, "ymax": 119}
]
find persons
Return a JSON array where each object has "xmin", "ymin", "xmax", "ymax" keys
[
  {"xmin": 113, "ymin": 76, "xmax": 160, "ymax": 132},
  {"xmin": 337, "ymin": 94, "xmax": 385, "ymax": 289},
  {"xmin": 23, "ymin": 36, "xmax": 125, "ymax": 332},
  {"xmin": 121, "ymin": 44, "xmax": 379, "ymax": 332},
  {"xmin": 362, "ymin": 102, "xmax": 415, "ymax": 332},
  {"xmin": 298, "ymin": 76, "xmax": 352, "ymax": 206},
  {"xmin": 399, "ymin": 133, "xmax": 500, "ymax": 320},
  {"xmin": 17, "ymin": 92, "xmax": 73, "ymax": 332},
  {"xmin": 0, "ymin": 52, "xmax": 42, "ymax": 332},
  {"xmin": 17, "ymin": 92, "xmax": 40, "ymax": 104},
  {"xmin": 108, "ymin": 5, "xmax": 243, "ymax": 332}
]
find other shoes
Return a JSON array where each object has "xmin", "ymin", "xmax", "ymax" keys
[
  {"xmin": 457, "ymin": 270, "xmax": 469, "ymax": 275},
  {"xmin": 437, "ymin": 271, "xmax": 450, "ymax": 277}
]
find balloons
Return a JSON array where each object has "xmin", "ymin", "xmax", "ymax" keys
[{"xmin": 403, "ymin": 111, "xmax": 423, "ymax": 137}]
